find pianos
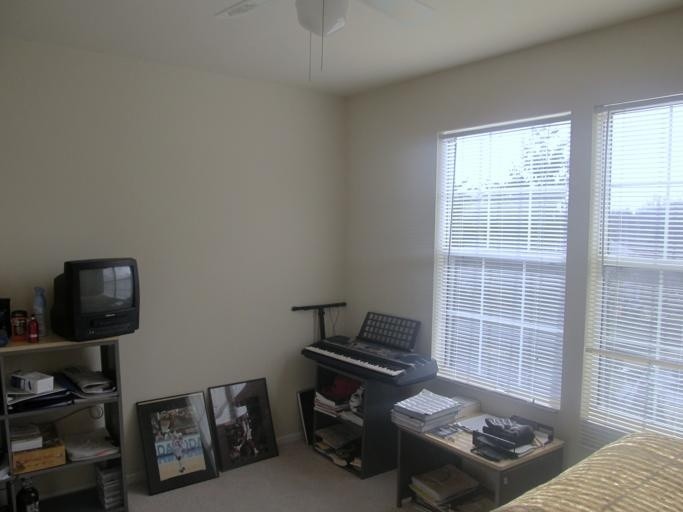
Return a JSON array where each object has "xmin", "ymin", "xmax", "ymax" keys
[{"xmin": 300, "ymin": 334, "xmax": 439, "ymax": 387}]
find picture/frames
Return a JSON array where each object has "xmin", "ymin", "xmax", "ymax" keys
[{"xmin": 133, "ymin": 376, "xmax": 281, "ymax": 496}]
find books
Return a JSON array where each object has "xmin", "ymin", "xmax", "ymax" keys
[
  {"xmin": 9, "ymin": 422, "xmax": 43, "ymax": 452},
  {"xmin": 391, "ymin": 388, "xmax": 477, "ymax": 432},
  {"xmin": 405, "ymin": 462, "xmax": 495, "ymax": 512},
  {"xmin": 59, "ymin": 367, "xmax": 117, "ymax": 401},
  {"xmin": 314, "ymin": 392, "xmax": 364, "ymax": 426}
]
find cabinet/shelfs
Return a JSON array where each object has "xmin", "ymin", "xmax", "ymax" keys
[
  {"xmin": 1, "ymin": 335, "xmax": 132, "ymax": 511},
  {"xmin": 309, "ymin": 361, "xmax": 420, "ymax": 481}
]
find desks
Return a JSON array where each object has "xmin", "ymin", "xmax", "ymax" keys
[{"xmin": 394, "ymin": 392, "xmax": 567, "ymax": 511}]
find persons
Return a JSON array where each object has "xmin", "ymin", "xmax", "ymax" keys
[{"xmin": 168, "ymin": 426, "xmax": 186, "ymax": 473}]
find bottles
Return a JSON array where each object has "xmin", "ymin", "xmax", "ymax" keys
[
  {"xmin": 11, "ymin": 286, "xmax": 45, "ymax": 343},
  {"xmin": 14, "ymin": 473, "xmax": 39, "ymax": 512}
]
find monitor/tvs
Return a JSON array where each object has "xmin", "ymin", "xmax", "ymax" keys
[{"xmin": 49, "ymin": 257, "xmax": 140, "ymax": 342}]
